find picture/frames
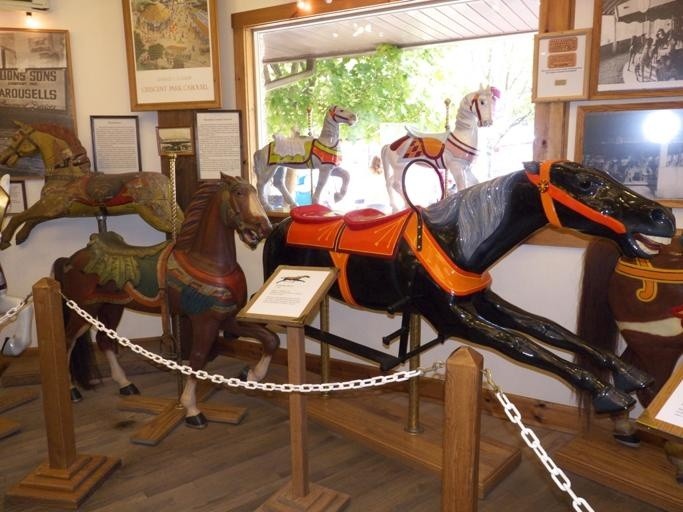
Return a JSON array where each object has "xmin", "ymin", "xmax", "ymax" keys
[
  {"xmin": 122, "ymin": 0, "xmax": 222, "ymax": 112},
  {"xmin": 0, "ymin": 28, "xmax": 78, "ymax": 180},
  {"xmin": 90, "ymin": 115, "xmax": 142, "ymax": 172},
  {"xmin": 194, "ymin": 109, "xmax": 244, "ymax": 183},
  {"xmin": 235, "ymin": 264, "xmax": 340, "ymax": 328},
  {"xmin": 6, "ymin": 180, "xmax": 27, "ymax": 217},
  {"xmin": 634, "ymin": 362, "xmax": 683, "ymax": 445},
  {"xmin": 532, "ymin": 0, "xmax": 683, "ymax": 208},
  {"xmin": 156, "ymin": 126, "xmax": 196, "ymax": 156}
]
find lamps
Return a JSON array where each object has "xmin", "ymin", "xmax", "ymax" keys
[{"xmin": 25, "ymin": 12, "xmax": 34, "ymax": 26}]
